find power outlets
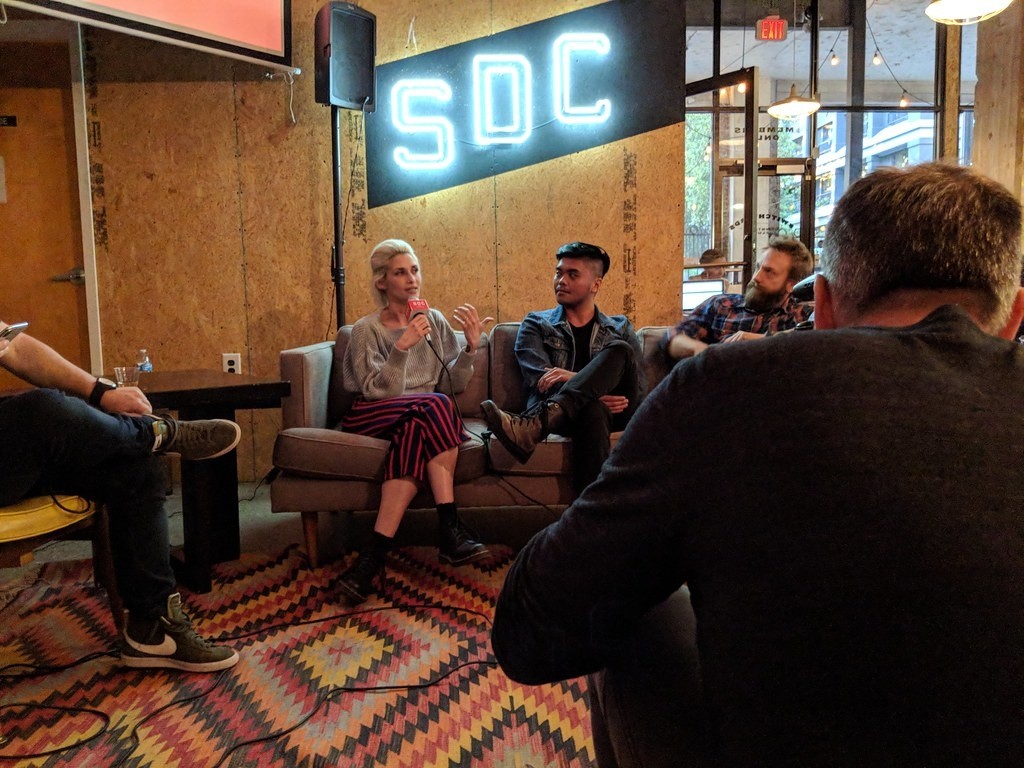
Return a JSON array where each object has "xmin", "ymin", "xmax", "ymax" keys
[{"xmin": 222, "ymin": 353, "xmax": 241, "ymax": 374}]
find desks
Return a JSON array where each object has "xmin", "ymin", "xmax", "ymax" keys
[{"xmin": 102, "ymin": 369, "xmax": 291, "ymax": 566}]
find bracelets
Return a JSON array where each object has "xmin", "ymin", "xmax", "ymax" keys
[
  {"xmin": 765, "ymin": 330, "xmax": 774, "ymax": 337},
  {"xmin": 87, "ymin": 378, "xmax": 117, "ymax": 409}
]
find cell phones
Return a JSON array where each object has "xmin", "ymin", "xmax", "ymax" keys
[{"xmin": 0, "ymin": 323, "xmax": 29, "ymax": 343}]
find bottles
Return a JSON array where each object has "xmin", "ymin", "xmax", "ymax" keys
[{"xmin": 137, "ymin": 349, "xmax": 152, "ymax": 373}]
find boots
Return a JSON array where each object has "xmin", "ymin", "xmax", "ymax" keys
[
  {"xmin": 339, "ymin": 538, "xmax": 387, "ymax": 602},
  {"xmin": 480, "ymin": 400, "xmax": 562, "ymax": 465},
  {"xmin": 436, "ymin": 501, "xmax": 492, "ymax": 566}
]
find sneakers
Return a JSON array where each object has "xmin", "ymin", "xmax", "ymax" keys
[
  {"xmin": 142, "ymin": 413, "xmax": 243, "ymax": 461},
  {"xmin": 121, "ymin": 590, "xmax": 240, "ymax": 673}
]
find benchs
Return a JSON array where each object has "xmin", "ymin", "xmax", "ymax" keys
[{"xmin": 271, "ymin": 323, "xmax": 679, "ymax": 569}]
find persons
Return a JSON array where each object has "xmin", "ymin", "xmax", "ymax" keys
[
  {"xmin": 660, "ymin": 236, "xmax": 814, "ymax": 359},
  {"xmin": 688, "ymin": 249, "xmax": 730, "ymax": 293},
  {"xmin": 487, "ymin": 164, "xmax": 1024, "ymax": 768},
  {"xmin": 336, "ymin": 240, "xmax": 494, "ymax": 601},
  {"xmin": 481, "ymin": 242, "xmax": 647, "ymax": 506},
  {"xmin": 1, "ymin": 320, "xmax": 243, "ymax": 670}
]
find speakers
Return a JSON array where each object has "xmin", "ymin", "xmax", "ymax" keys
[{"xmin": 314, "ymin": 1, "xmax": 377, "ymax": 112}]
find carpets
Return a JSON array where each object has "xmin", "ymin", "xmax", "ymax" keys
[{"xmin": 0, "ymin": 547, "xmax": 598, "ymax": 768}]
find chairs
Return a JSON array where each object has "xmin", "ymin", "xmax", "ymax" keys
[{"xmin": 0, "ymin": 494, "xmax": 126, "ymax": 633}]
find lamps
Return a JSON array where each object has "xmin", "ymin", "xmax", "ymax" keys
[
  {"xmin": 766, "ymin": 0, "xmax": 820, "ymax": 120},
  {"xmin": 924, "ymin": 0, "xmax": 1014, "ymax": 26}
]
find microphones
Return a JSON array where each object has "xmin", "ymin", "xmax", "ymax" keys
[{"xmin": 404, "ymin": 296, "xmax": 433, "ymax": 346}]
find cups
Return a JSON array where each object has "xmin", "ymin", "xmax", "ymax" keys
[{"xmin": 114, "ymin": 367, "xmax": 140, "ymax": 388}]
